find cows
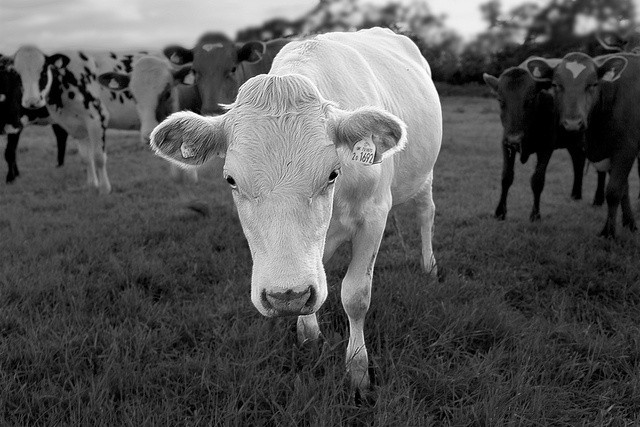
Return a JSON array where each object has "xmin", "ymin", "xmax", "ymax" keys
[
  {"xmin": 162, "ymin": 32, "xmax": 302, "ymax": 115},
  {"xmin": 149, "ymin": 26, "xmax": 443, "ymax": 392},
  {"xmin": 483, "ymin": 56, "xmax": 604, "ymax": 222},
  {"xmin": 527, "ymin": 52, "xmax": 640, "ymax": 239},
  {"xmin": 0, "ymin": 53, "xmax": 69, "ymax": 184},
  {"xmin": 154, "ymin": 80, "xmax": 202, "ymax": 122},
  {"xmin": 0, "ymin": 46, "xmax": 175, "ymax": 193}
]
